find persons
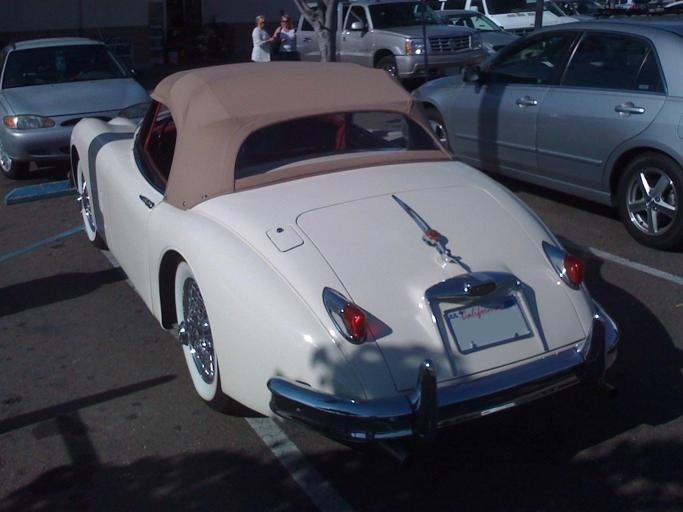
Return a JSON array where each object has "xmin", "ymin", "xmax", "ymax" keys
[
  {"xmin": 249, "ymin": 13, "xmax": 275, "ymax": 62},
  {"xmin": 272, "ymin": 13, "xmax": 297, "ymax": 61}
]
